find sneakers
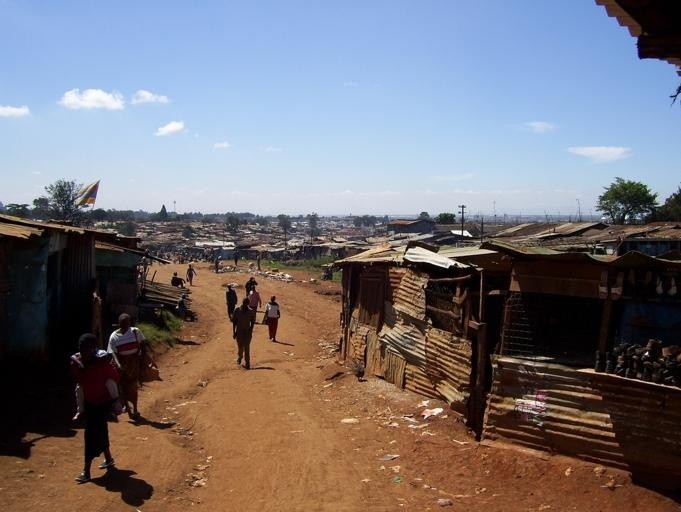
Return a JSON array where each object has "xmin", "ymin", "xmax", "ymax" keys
[
  {"xmin": 236, "ymin": 359, "xmax": 250, "ymax": 369},
  {"xmin": 98, "ymin": 458, "xmax": 114, "ymax": 468},
  {"xmin": 114, "ymin": 402, "xmax": 140, "ymax": 424},
  {"xmin": 594, "ymin": 338, "xmax": 681, "ymax": 389},
  {"xmin": 73, "ymin": 412, "xmax": 84, "ymax": 420},
  {"xmin": 269, "ymin": 336, "xmax": 275, "ymax": 342},
  {"xmin": 75, "ymin": 471, "xmax": 91, "ymax": 482}
]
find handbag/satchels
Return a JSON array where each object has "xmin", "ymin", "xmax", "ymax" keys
[
  {"xmin": 261, "ymin": 310, "xmax": 268, "ymax": 324},
  {"xmin": 138, "ymin": 352, "xmax": 159, "ymax": 381}
]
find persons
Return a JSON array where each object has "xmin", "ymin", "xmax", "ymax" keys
[
  {"xmin": 144, "ymin": 245, "xmax": 240, "ymax": 289},
  {"xmin": 232, "ymin": 297, "xmax": 255, "ymax": 370},
  {"xmin": 257, "ymin": 253, "xmax": 261, "ymax": 270},
  {"xmin": 107, "ymin": 313, "xmax": 159, "ymax": 423},
  {"xmin": 245, "ymin": 276, "xmax": 258, "ymax": 296},
  {"xmin": 226, "ymin": 284, "xmax": 239, "ymax": 319},
  {"xmin": 321, "ymin": 266, "xmax": 333, "ymax": 281},
  {"xmin": 71, "ymin": 334, "xmax": 126, "ymax": 421},
  {"xmin": 261, "ymin": 295, "xmax": 281, "ymax": 341},
  {"xmin": 249, "ymin": 285, "xmax": 263, "ymax": 324},
  {"xmin": 69, "ymin": 334, "xmax": 122, "ymax": 483}
]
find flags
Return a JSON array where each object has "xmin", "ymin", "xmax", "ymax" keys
[{"xmin": 75, "ymin": 178, "xmax": 101, "ymax": 206}]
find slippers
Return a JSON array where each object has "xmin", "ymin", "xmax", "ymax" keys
[{"xmin": 597, "ymin": 267, "xmax": 678, "ymax": 301}]
